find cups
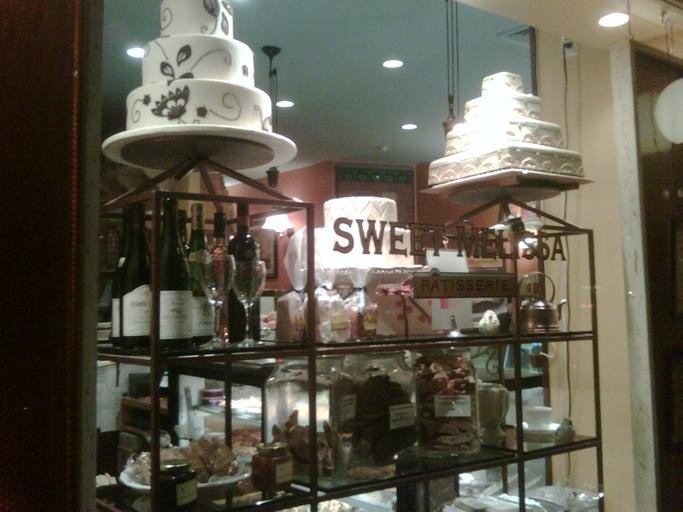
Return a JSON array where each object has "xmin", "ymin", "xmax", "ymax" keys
[
  {"xmin": 509, "ymin": 270, "xmax": 567, "ymax": 336},
  {"xmin": 524, "ymin": 405, "xmax": 554, "ymax": 428}
]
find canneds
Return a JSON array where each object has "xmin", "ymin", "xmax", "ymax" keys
[
  {"xmin": 153, "ymin": 459, "xmax": 198, "ymax": 512},
  {"xmin": 250, "ymin": 442, "xmax": 294, "ymax": 490}
]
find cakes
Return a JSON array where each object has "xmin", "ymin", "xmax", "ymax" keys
[
  {"xmin": 426, "ymin": 71, "xmax": 584, "ymax": 186},
  {"xmin": 313, "ymin": 195, "xmax": 412, "ymax": 267},
  {"xmin": 123, "ymin": 0, "xmax": 274, "ymax": 130}
]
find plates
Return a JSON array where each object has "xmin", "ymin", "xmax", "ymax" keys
[
  {"xmin": 117, "ymin": 460, "xmax": 253, "ymax": 492},
  {"xmin": 514, "ymin": 421, "xmax": 560, "ymax": 434}
]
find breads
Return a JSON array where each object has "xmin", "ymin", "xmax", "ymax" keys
[{"xmin": 276, "ymin": 296, "xmax": 351, "ymax": 344}]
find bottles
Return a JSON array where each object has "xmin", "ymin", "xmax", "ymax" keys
[
  {"xmin": 107, "ymin": 198, "xmax": 263, "ymax": 352},
  {"xmin": 415, "ymin": 348, "xmax": 482, "ymax": 457},
  {"xmin": 199, "ymin": 389, "xmax": 224, "ymax": 407}
]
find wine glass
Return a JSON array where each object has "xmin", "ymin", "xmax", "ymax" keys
[{"xmin": 232, "ymin": 260, "xmax": 267, "ymax": 349}]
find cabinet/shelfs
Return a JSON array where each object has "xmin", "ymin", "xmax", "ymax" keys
[{"xmin": 96, "ymin": 172, "xmax": 607, "ymax": 507}]
